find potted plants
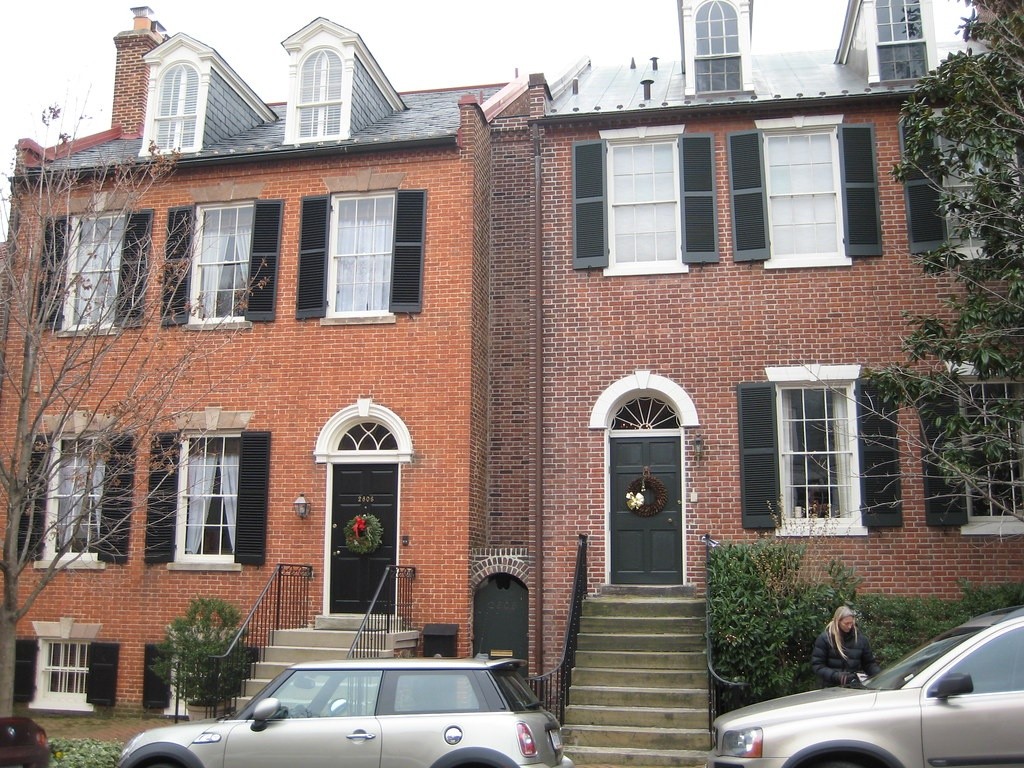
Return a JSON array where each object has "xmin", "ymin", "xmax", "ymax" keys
[
  {"xmin": 708, "ymin": 541, "xmax": 803, "ymax": 717},
  {"xmin": 148, "ymin": 595, "xmax": 254, "ymax": 722}
]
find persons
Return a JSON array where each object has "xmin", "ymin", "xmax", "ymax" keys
[{"xmin": 811, "ymin": 606, "xmax": 880, "ymax": 690}]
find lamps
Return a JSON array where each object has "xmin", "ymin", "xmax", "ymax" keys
[
  {"xmin": 294, "ymin": 492, "xmax": 311, "ymax": 518},
  {"xmin": 691, "ymin": 433, "xmax": 705, "ymax": 467}
]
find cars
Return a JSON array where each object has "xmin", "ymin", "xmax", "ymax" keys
[
  {"xmin": 0, "ymin": 716, "xmax": 50, "ymax": 768},
  {"xmin": 703, "ymin": 602, "xmax": 1023, "ymax": 768},
  {"xmin": 117, "ymin": 654, "xmax": 577, "ymax": 768}
]
